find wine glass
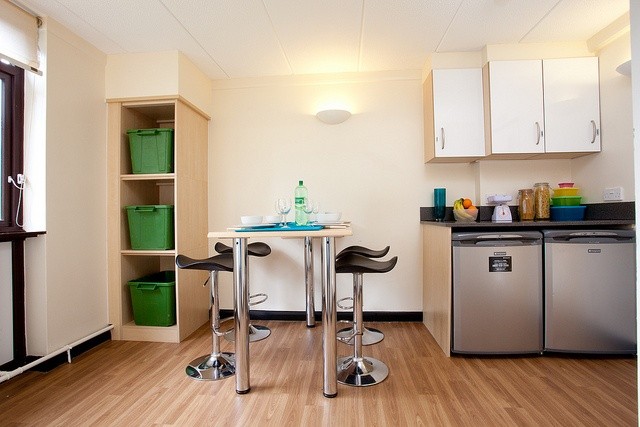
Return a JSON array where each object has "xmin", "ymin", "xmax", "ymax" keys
[
  {"xmin": 274, "ymin": 201, "xmax": 284, "ymax": 226},
  {"xmin": 313, "ymin": 201, "xmax": 319, "ymax": 223},
  {"xmin": 278, "ymin": 197, "xmax": 291, "ymax": 228},
  {"xmin": 304, "ymin": 198, "xmax": 314, "ymax": 226}
]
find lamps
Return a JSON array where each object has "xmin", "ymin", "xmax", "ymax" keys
[{"xmin": 315, "ymin": 109, "xmax": 352, "ymax": 124}]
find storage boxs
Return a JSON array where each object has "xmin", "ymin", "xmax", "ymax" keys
[
  {"xmin": 122, "ymin": 203, "xmax": 173, "ymax": 251},
  {"xmin": 125, "ymin": 125, "xmax": 175, "ymax": 174},
  {"xmin": 128, "ymin": 270, "xmax": 177, "ymax": 327}
]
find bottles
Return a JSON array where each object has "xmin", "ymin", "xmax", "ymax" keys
[{"xmin": 294, "ymin": 180, "xmax": 308, "ymax": 226}]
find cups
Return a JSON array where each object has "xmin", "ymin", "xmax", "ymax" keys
[{"xmin": 434, "ymin": 188, "xmax": 446, "ymax": 222}]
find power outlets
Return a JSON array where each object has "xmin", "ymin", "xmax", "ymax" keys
[{"xmin": 602, "ymin": 185, "xmax": 624, "ymax": 202}]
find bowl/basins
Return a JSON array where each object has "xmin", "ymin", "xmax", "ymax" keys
[
  {"xmin": 265, "ymin": 216, "xmax": 284, "ymax": 224},
  {"xmin": 559, "ymin": 183, "xmax": 574, "ymax": 187},
  {"xmin": 549, "ymin": 205, "xmax": 587, "ymax": 220},
  {"xmin": 551, "ymin": 196, "xmax": 582, "ymax": 206},
  {"xmin": 553, "ymin": 188, "xmax": 579, "ymax": 196},
  {"xmin": 241, "ymin": 215, "xmax": 263, "ymax": 224},
  {"xmin": 316, "ymin": 211, "xmax": 342, "ymax": 223},
  {"xmin": 452, "ymin": 209, "xmax": 479, "ymax": 222}
]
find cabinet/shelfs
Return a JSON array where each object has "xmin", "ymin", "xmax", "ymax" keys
[
  {"xmin": 420, "ymin": 225, "xmax": 545, "ymax": 357},
  {"xmin": 484, "ymin": 60, "xmax": 545, "ymax": 161},
  {"xmin": 424, "ymin": 67, "xmax": 485, "ymax": 165},
  {"xmin": 543, "ymin": 56, "xmax": 604, "ymax": 162},
  {"xmin": 107, "ymin": 94, "xmax": 213, "ymax": 346},
  {"xmin": 544, "ymin": 228, "xmax": 637, "ymax": 358}
]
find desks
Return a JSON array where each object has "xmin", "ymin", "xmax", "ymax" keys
[{"xmin": 206, "ymin": 220, "xmax": 354, "ymax": 399}]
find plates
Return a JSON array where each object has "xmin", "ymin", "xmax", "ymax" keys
[
  {"xmin": 233, "ymin": 224, "xmax": 276, "ymax": 227},
  {"xmin": 315, "ymin": 222, "xmax": 345, "ymax": 223}
]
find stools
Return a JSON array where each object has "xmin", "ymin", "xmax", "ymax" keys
[
  {"xmin": 334, "ymin": 250, "xmax": 399, "ymax": 388},
  {"xmin": 214, "ymin": 240, "xmax": 274, "ymax": 344},
  {"xmin": 175, "ymin": 252, "xmax": 237, "ymax": 381},
  {"xmin": 337, "ymin": 245, "xmax": 392, "ymax": 348}
]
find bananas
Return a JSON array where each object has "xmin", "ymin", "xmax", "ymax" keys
[{"xmin": 453, "ymin": 198, "xmax": 472, "ymax": 219}]
